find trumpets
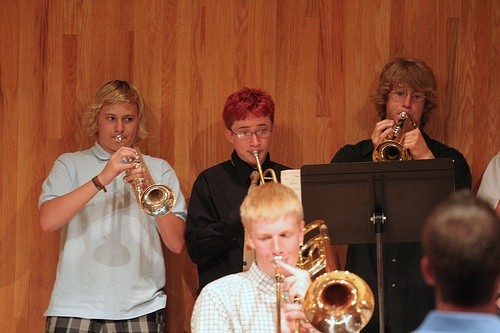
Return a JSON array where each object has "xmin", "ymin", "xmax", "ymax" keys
[
  {"xmin": 252, "ymin": 150, "xmax": 279, "ymax": 186},
  {"xmin": 370, "ymin": 112, "xmax": 422, "ymax": 163},
  {"xmin": 117, "ymin": 133, "xmax": 174, "ymax": 217}
]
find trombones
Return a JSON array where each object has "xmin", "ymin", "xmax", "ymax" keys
[{"xmin": 274, "ymin": 218, "xmax": 375, "ymax": 333}]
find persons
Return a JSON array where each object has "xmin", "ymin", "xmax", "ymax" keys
[
  {"xmin": 191, "ymin": 182, "xmax": 323, "ymax": 333},
  {"xmin": 185, "ymin": 86, "xmax": 295, "ymax": 297},
  {"xmin": 37, "ymin": 80, "xmax": 188, "ymax": 333},
  {"xmin": 411, "ymin": 190, "xmax": 500, "ymax": 333},
  {"xmin": 330, "ymin": 57, "xmax": 472, "ymax": 333}
]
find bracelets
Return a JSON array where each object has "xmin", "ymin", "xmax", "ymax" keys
[{"xmin": 92, "ymin": 175, "xmax": 107, "ymax": 193}]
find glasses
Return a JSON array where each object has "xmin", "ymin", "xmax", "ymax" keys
[
  {"xmin": 229, "ymin": 124, "xmax": 272, "ymax": 140},
  {"xmin": 389, "ymin": 89, "xmax": 427, "ymax": 102}
]
